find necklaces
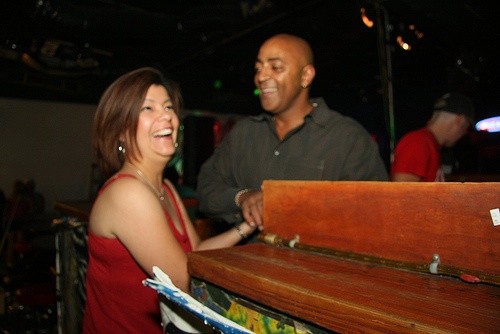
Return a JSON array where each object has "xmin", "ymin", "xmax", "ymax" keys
[{"xmin": 125, "ymin": 162, "xmax": 169, "ymax": 204}]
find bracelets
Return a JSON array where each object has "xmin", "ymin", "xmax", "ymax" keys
[{"xmin": 233, "ymin": 222, "xmax": 249, "ymax": 241}]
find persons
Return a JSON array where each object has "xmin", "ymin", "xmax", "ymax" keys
[
  {"xmin": 388, "ymin": 87, "xmax": 478, "ymax": 184},
  {"xmin": 194, "ymin": 33, "xmax": 387, "ymax": 225},
  {"xmin": 72, "ymin": 63, "xmax": 263, "ymax": 333}
]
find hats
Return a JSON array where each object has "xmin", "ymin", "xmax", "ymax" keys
[{"xmin": 437, "ymin": 93, "xmax": 477, "ymax": 131}]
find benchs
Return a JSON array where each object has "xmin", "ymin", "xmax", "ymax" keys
[{"xmin": 188, "ymin": 183, "xmax": 496, "ymax": 334}]
